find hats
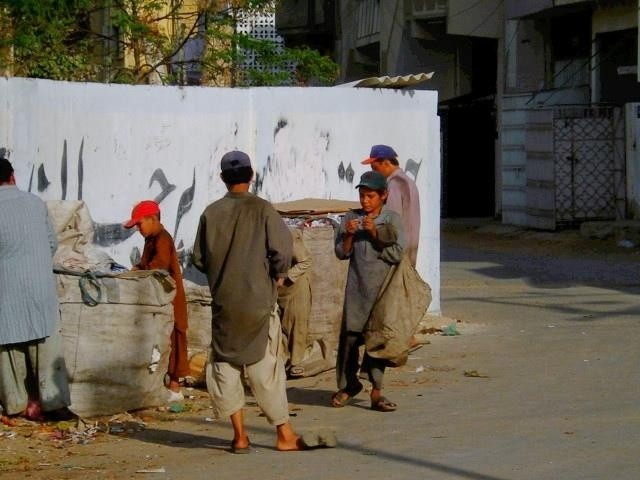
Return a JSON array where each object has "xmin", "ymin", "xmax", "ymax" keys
[
  {"xmin": 221, "ymin": 150, "xmax": 252, "ymax": 171},
  {"xmin": 360, "ymin": 145, "xmax": 397, "ymax": 165},
  {"xmin": 355, "ymin": 171, "xmax": 388, "ymax": 191},
  {"xmin": 124, "ymin": 200, "xmax": 160, "ymax": 230}
]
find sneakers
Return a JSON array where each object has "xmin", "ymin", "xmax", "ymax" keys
[
  {"xmin": 43, "ymin": 408, "xmax": 81, "ymax": 421},
  {"xmin": 168, "ymin": 388, "xmax": 186, "ymax": 402}
]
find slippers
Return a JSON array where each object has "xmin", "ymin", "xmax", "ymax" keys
[
  {"xmin": 300, "ymin": 428, "xmax": 337, "ymax": 450},
  {"xmin": 371, "ymin": 395, "xmax": 397, "ymax": 412},
  {"xmin": 231, "ymin": 434, "xmax": 250, "ymax": 454},
  {"xmin": 331, "ymin": 382, "xmax": 363, "ymax": 407}
]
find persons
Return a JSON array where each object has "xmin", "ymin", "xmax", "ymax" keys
[
  {"xmin": 193, "ymin": 151, "xmax": 313, "ymax": 451},
  {"xmin": 330, "ymin": 146, "xmax": 421, "ymax": 411},
  {"xmin": 0, "ymin": 159, "xmax": 78, "ymax": 420},
  {"xmin": 124, "ymin": 201, "xmax": 190, "ymax": 402}
]
